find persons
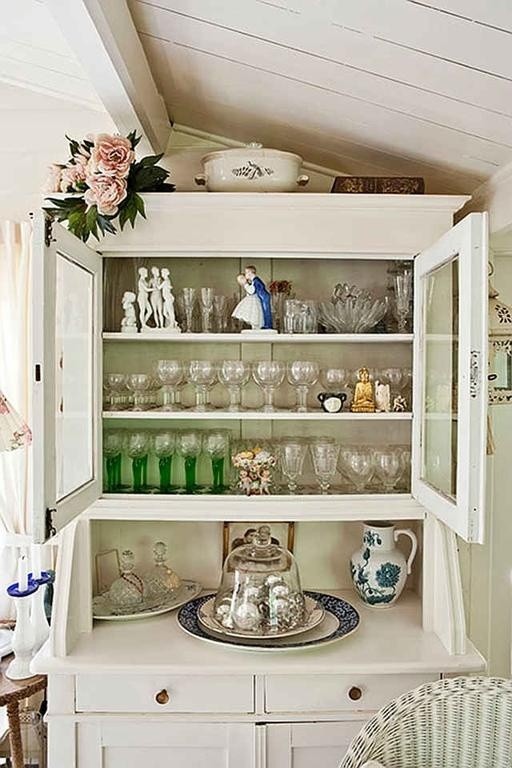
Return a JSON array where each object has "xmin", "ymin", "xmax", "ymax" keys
[
  {"xmin": 256, "ymin": 470, "xmax": 273, "ymax": 495},
  {"xmin": 237, "ymin": 471, "xmax": 253, "ymax": 498},
  {"xmin": 234, "ymin": 525, "xmax": 281, "ymax": 565},
  {"xmin": 350, "ymin": 366, "xmax": 374, "ymax": 405},
  {"xmin": 245, "ymin": 266, "xmax": 275, "ymax": 330},
  {"xmin": 117, "ymin": 292, "xmax": 136, "ymax": 327},
  {"xmin": 134, "ymin": 266, "xmax": 178, "ymax": 328},
  {"xmin": 231, "ymin": 273, "xmax": 264, "ymax": 328}
]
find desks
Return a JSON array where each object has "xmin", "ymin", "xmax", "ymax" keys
[{"xmin": 0, "ymin": 652, "xmax": 47, "ymax": 768}]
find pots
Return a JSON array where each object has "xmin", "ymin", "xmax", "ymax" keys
[{"xmin": 192, "ymin": 141, "xmax": 309, "ymax": 193}]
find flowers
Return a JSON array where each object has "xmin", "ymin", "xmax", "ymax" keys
[{"xmin": 41, "ymin": 130, "xmax": 175, "ymax": 244}]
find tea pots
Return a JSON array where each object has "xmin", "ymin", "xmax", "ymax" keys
[{"xmin": 348, "ymin": 520, "xmax": 418, "ymax": 611}]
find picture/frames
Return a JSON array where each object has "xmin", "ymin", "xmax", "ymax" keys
[{"xmin": 224, "ymin": 522, "xmax": 294, "ymax": 573}]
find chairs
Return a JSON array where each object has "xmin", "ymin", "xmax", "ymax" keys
[{"xmin": 339, "ymin": 676, "xmax": 512, "ymax": 768}]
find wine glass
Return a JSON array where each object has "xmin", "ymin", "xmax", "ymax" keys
[
  {"xmin": 174, "ymin": 283, "xmax": 246, "ymax": 335},
  {"xmin": 389, "ymin": 269, "xmax": 415, "ymax": 335},
  {"xmin": 105, "ymin": 358, "xmax": 414, "ymax": 415},
  {"xmin": 105, "ymin": 421, "xmax": 411, "ymax": 495}
]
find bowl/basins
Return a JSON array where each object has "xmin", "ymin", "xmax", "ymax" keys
[{"xmin": 314, "ymin": 296, "xmax": 388, "ymax": 334}]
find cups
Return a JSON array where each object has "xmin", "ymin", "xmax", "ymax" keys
[{"xmin": 283, "ymin": 297, "xmax": 319, "ymax": 334}]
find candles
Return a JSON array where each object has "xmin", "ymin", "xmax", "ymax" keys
[
  {"xmin": 30, "ymin": 544, "xmax": 42, "ymax": 580},
  {"xmin": 17, "ymin": 554, "xmax": 28, "ymax": 593}
]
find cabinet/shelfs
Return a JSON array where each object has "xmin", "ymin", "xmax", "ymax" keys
[{"xmin": 29, "ymin": 192, "xmax": 488, "ymax": 768}]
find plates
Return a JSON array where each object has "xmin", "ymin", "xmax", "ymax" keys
[
  {"xmin": 90, "ymin": 580, "xmax": 203, "ymax": 623},
  {"xmin": 175, "ymin": 590, "xmax": 361, "ymax": 653}
]
